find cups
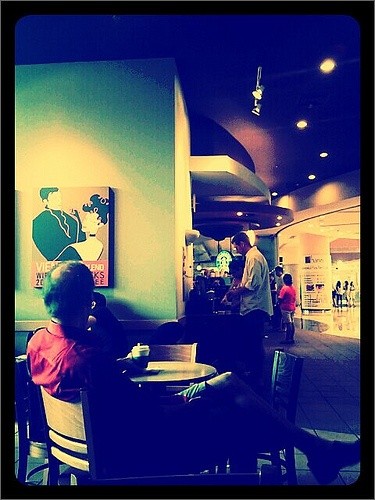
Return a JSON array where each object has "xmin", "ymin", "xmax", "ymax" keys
[{"xmin": 131, "ymin": 345, "xmax": 150, "ymax": 360}]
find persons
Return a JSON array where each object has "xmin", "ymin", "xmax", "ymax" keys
[
  {"xmin": 219, "ymin": 232, "xmax": 274, "ymax": 386},
  {"xmin": 275, "ymin": 266, "xmax": 297, "ymax": 345},
  {"xmin": 24, "ymin": 260, "xmax": 363, "ymax": 485},
  {"xmin": 84, "ymin": 291, "xmax": 129, "ymax": 361},
  {"xmin": 331, "ymin": 280, "xmax": 356, "ymax": 308}
]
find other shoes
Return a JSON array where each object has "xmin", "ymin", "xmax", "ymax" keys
[{"xmin": 307, "ymin": 438, "xmax": 361, "ymax": 483}]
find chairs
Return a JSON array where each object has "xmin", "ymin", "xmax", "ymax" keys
[{"xmin": 17, "ymin": 323, "xmax": 305, "ymax": 485}]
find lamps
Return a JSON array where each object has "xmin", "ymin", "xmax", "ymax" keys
[{"xmin": 252, "ymin": 67, "xmax": 264, "ymax": 115}]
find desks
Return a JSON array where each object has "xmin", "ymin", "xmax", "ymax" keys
[{"xmin": 130, "ymin": 361, "xmax": 218, "ymax": 393}]
